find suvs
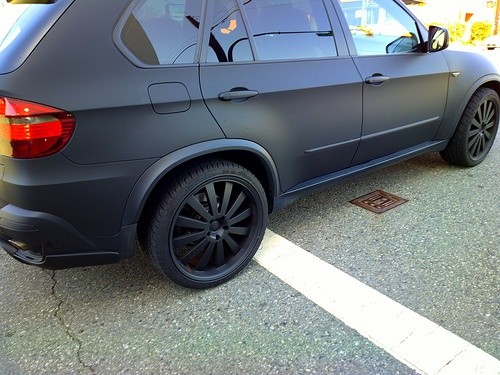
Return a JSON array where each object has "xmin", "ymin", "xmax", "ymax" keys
[{"xmin": 0, "ymin": 0, "xmax": 500, "ymax": 290}]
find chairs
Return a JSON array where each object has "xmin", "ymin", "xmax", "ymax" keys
[{"xmin": 181, "ymin": 3, "xmax": 311, "ymax": 33}]
